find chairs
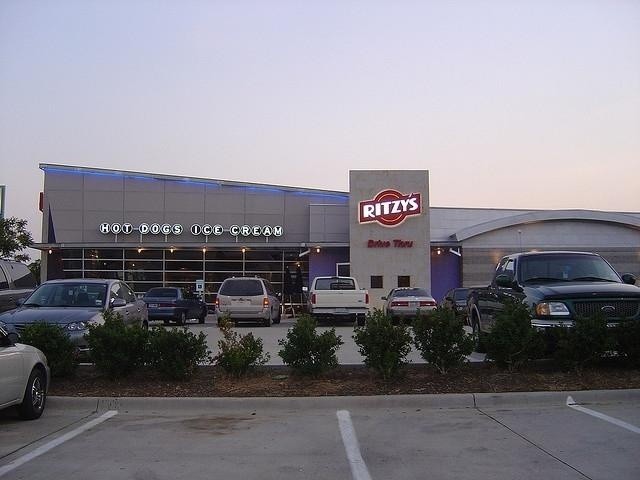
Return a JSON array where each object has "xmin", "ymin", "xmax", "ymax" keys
[{"xmin": 53, "ymin": 287, "xmax": 106, "ymax": 304}]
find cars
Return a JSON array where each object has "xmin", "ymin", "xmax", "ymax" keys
[
  {"xmin": 0, "ymin": 260, "xmax": 208, "ymax": 419},
  {"xmin": 381, "ymin": 286, "xmax": 469, "ymax": 320}
]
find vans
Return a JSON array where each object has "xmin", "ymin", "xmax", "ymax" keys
[{"xmin": 215, "ymin": 276, "xmax": 283, "ymax": 327}]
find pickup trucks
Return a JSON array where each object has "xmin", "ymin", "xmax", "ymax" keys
[
  {"xmin": 301, "ymin": 275, "xmax": 369, "ymax": 326},
  {"xmin": 468, "ymin": 250, "xmax": 639, "ymax": 353}
]
